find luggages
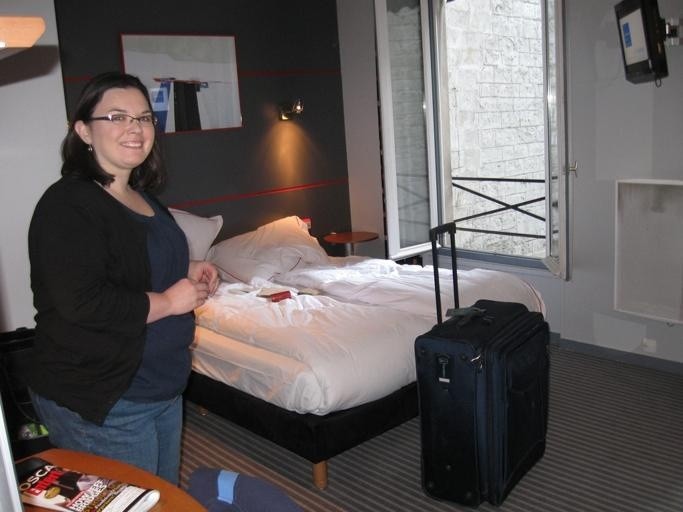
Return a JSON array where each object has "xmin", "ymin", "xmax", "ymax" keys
[
  {"xmin": 0, "ymin": 327, "xmax": 51, "ymax": 462},
  {"xmin": 414, "ymin": 221, "xmax": 550, "ymax": 509}
]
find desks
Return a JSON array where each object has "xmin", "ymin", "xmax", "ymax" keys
[
  {"xmin": 12, "ymin": 446, "xmax": 208, "ymax": 512},
  {"xmin": 324, "ymin": 231, "xmax": 378, "ymax": 256}
]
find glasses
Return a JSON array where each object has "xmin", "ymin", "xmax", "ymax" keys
[{"xmin": 88, "ymin": 113, "xmax": 160, "ymax": 127}]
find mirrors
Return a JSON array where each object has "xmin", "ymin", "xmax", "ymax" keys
[{"xmin": 118, "ymin": 31, "xmax": 245, "ymax": 138}]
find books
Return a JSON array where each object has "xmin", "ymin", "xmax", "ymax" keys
[
  {"xmin": 297, "ymin": 288, "xmax": 324, "ymax": 296},
  {"xmin": 254, "ymin": 286, "xmax": 291, "ymax": 297},
  {"xmin": 15, "ymin": 461, "xmax": 162, "ymax": 512},
  {"xmin": 270, "ymin": 291, "xmax": 292, "ymax": 302},
  {"xmin": 226, "ymin": 285, "xmax": 260, "ymax": 295}
]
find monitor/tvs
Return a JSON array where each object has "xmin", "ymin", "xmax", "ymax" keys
[{"xmin": 612, "ymin": 0, "xmax": 670, "ymax": 87}]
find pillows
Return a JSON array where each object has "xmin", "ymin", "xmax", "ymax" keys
[{"xmin": 166, "ymin": 207, "xmax": 329, "ymax": 289}]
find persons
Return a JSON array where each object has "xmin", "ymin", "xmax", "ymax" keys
[{"xmin": 25, "ymin": 69, "xmax": 222, "ymax": 490}]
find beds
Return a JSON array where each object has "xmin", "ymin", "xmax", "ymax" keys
[{"xmin": 179, "ymin": 255, "xmax": 546, "ymax": 490}]
